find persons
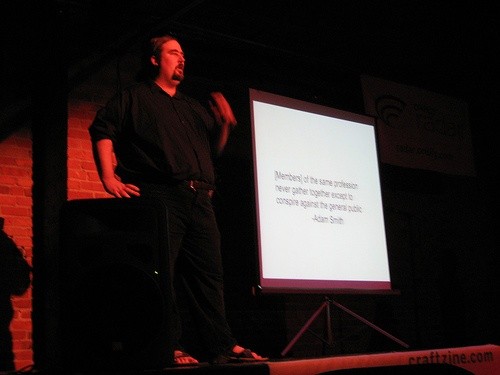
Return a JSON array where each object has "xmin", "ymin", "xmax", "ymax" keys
[{"xmin": 88, "ymin": 36, "xmax": 271, "ymax": 369}]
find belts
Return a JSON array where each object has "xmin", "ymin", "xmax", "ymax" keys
[{"xmin": 172, "ymin": 177, "xmax": 218, "ymax": 195}]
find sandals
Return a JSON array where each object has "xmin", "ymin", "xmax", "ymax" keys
[
  {"xmin": 213, "ymin": 343, "xmax": 268, "ymax": 365},
  {"xmin": 165, "ymin": 349, "xmax": 198, "ymax": 368}
]
